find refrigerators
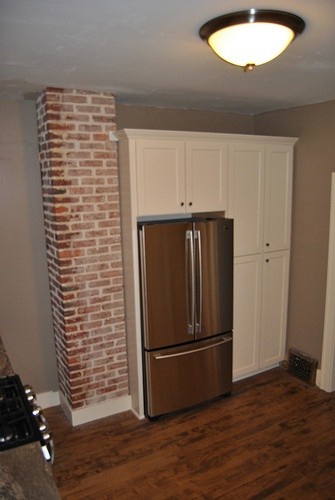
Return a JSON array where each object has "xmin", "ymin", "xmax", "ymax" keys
[{"xmin": 136, "ymin": 210, "xmax": 236, "ymax": 420}]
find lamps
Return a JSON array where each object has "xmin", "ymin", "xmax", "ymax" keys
[{"xmin": 198, "ymin": 9, "xmax": 305, "ymax": 71}]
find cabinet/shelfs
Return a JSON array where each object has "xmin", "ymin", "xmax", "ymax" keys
[{"xmin": 116, "ymin": 128, "xmax": 300, "ymax": 422}]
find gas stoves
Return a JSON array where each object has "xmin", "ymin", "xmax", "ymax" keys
[{"xmin": 0, "ymin": 370, "xmax": 49, "ymax": 454}]
